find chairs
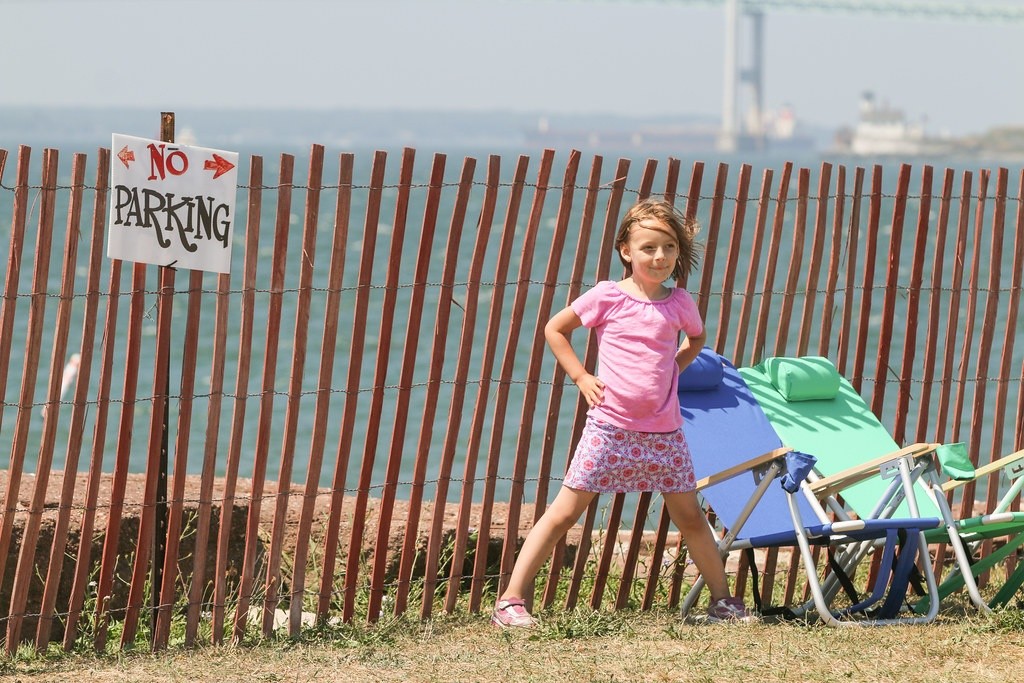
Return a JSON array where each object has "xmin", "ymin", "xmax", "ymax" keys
[
  {"xmin": 737, "ymin": 355, "xmax": 1023, "ymax": 614},
  {"xmin": 674, "ymin": 344, "xmax": 938, "ymax": 629}
]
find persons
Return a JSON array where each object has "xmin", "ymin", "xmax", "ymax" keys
[{"xmin": 490, "ymin": 200, "xmax": 760, "ymax": 630}]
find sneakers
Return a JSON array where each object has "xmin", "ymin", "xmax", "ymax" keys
[
  {"xmin": 490, "ymin": 597, "xmax": 541, "ymax": 629},
  {"xmin": 706, "ymin": 596, "xmax": 759, "ymax": 625}
]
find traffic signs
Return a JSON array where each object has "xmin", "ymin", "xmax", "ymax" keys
[{"xmin": 104, "ymin": 128, "xmax": 242, "ymax": 277}]
